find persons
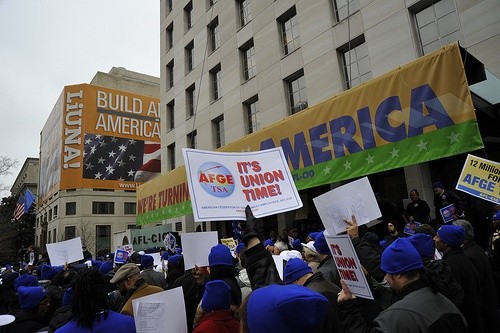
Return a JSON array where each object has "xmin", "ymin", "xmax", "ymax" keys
[
  {"xmin": 0, "ymin": 206, "xmax": 500, "ymax": 333},
  {"xmin": 405, "ymin": 189, "xmax": 431, "ymax": 224},
  {"xmin": 433, "ymin": 183, "xmax": 463, "ymax": 226}
]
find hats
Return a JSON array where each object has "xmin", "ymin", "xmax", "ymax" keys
[
  {"xmin": 236, "ymin": 243, "xmax": 246, "ymax": 253},
  {"xmin": 200, "ymin": 280, "xmax": 232, "ymax": 310},
  {"xmin": 141, "ymin": 255, "xmax": 153, "ymax": 267},
  {"xmin": 263, "ymin": 239, "xmax": 273, "ymax": 247},
  {"xmin": 13, "ymin": 274, "xmax": 38, "ymax": 291},
  {"xmin": 293, "ymin": 239, "xmax": 302, "ymax": 247},
  {"xmin": 313, "ymin": 231, "xmax": 331, "ymax": 255},
  {"xmin": 280, "ymin": 250, "xmax": 302, "ymax": 261},
  {"xmin": 300, "ymin": 241, "xmax": 317, "ymax": 253},
  {"xmin": 41, "ymin": 264, "xmax": 63, "ymax": 280},
  {"xmin": 99, "ymin": 261, "xmax": 112, "ymax": 272},
  {"xmin": 167, "ymin": 254, "xmax": 183, "ymax": 270},
  {"xmin": 308, "ymin": 232, "xmax": 319, "ymax": 240},
  {"xmin": 109, "ymin": 262, "xmax": 141, "ymax": 284},
  {"xmin": 380, "ymin": 237, "xmax": 424, "ymax": 274},
  {"xmin": 18, "ymin": 286, "xmax": 49, "ymax": 311},
  {"xmin": 283, "ymin": 258, "xmax": 314, "ymax": 284},
  {"xmin": 246, "ymin": 284, "xmax": 329, "ymax": 333},
  {"xmin": 209, "ymin": 244, "xmax": 233, "ymax": 266},
  {"xmin": 437, "ymin": 225, "xmax": 466, "ymax": 247},
  {"xmin": 408, "ymin": 233, "xmax": 435, "ymax": 255},
  {"xmin": 175, "ymin": 248, "xmax": 182, "ymax": 253},
  {"xmin": 162, "ymin": 252, "xmax": 168, "ymax": 259}
]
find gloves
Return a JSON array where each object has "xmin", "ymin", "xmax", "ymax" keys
[{"xmin": 236, "ymin": 205, "xmax": 263, "ymax": 246}]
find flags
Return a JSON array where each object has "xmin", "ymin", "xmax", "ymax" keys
[
  {"xmin": 25, "ymin": 189, "xmax": 35, "ymax": 213},
  {"xmin": 13, "ymin": 194, "xmax": 26, "ymax": 220}
]
